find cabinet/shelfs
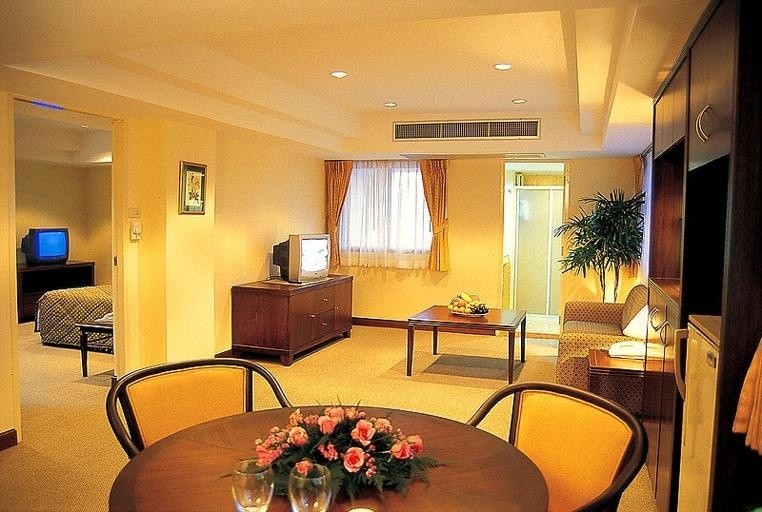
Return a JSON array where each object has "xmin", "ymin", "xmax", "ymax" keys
[
  {"xmin": 641, "ymin": 0, "xmax": 762, "ymax": 509},
  {"xmin": 230, "ymin": 273, "xmax": 353, "ymax": 367}
]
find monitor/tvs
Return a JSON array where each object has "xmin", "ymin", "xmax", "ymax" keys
[
  {"xmin": 21, "ymin": 228, "xmax": 70, "ymax": 266},
  {"xmin": 272, "ymin": 233, "xmax": 332, "ymax": 284}
]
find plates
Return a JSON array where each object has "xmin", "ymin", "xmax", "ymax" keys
[{"xmin": 447, "ymin": 307, "xmax": 490, "ymax": 317}]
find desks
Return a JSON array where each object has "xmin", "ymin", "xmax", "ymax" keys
[
  {"xmin": 73, "ymin": 321, "xmax": 113, "ymax": 377},
  {"xmin": 17, "ymin": 261, "xmax": 96, "ymax": 324}
]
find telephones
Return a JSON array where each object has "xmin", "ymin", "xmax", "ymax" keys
[
  {"xmin": 648, "ymin": 343, "xmax": 669, "ymax": 361},
  {"xmin": 608, "ymin": 341, "xmax": 646, "ymax": 361}
]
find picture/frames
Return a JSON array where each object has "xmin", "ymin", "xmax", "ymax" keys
[{"xmin": 178, "ymin": 161, "xmax": 208, "ymax": 215}]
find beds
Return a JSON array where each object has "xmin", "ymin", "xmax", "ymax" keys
[{"xmin": 38, "ymin": 284, "xmax": 113, "ymax": 355}]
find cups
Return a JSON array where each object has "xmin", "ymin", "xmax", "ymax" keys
[
  {"xmin": 230, "ymin": 460, "xmax": 273, "ymax": 511},
  {"xmin": 289, "ymin": 464, "xmax": 330, "ymax": 512}
]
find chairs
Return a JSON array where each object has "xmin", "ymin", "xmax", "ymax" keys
[
  {"xmin": 464, "ymin": 381, "xmax": 648, "ymax": 511},
  {"xmin": 106, "ymin": 359, "xmax": 295, "ymax": 460}
]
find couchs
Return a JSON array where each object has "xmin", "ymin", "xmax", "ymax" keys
[{"xmin": 556, "ymin": 284, "xmax": 649, "ymax": 417}]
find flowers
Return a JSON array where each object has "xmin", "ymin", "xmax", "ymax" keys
[{"xmin": 254, "ymin": 406, "xmax": 434, "ymax": 500}]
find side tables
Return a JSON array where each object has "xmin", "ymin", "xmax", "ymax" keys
[{"xmin": 588, "ymin": 348, "xmax": 676, "ymax": 421}]
